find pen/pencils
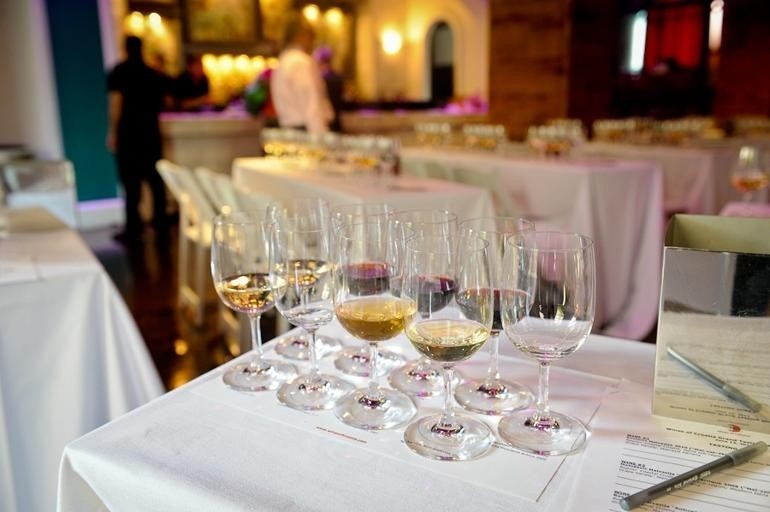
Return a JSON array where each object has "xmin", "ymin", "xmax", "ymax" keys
[
  {"xmin": 665, "ymin": 344, "xmax": 766, "ymax": 414},
  {"xmin": 620, "ymin": 441, "xmax": 768, "ymax": 510}
]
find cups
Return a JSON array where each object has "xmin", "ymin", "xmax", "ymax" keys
[{"xmin": 259, "ymin": 128, "xmax": 397, "ymax": 173}]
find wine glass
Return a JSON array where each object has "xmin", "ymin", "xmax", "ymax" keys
[
  {"xmin": 264, "ymin": 200, "xmax": 531, "ymax": 465},
  {"xmin": 211, "ymin": 202, "xmax": 290, "ymax": 391},
  {"xmin": 502, "ymin": 227, "xmax": 596, "ymax": 461},
  {"xmin": 731, "ymin": 147, "xmax": 768, "ymax": 204}
]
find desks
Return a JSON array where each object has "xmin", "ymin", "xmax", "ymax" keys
[
  {"xmin": 64, "ymin": 307, "xmax": 767, "ymax": 512},
  {"xmin": 2, "ymin": 132, "xmax": 769, "ymax": 512},
  {"xmin": 1, "ymin": 140, "xmax": 101, "ymax": 512},
  {"xmin": 157, "ymin": 134, "xmax": 769, "ymax": 352}
]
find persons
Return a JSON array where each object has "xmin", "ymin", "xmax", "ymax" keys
[
  {"xmin": 105, "ymin": 34, "xmax": 210, "ymax": 248},
  {"xmin": 268, "ymin": 10, "xmax": 344, "ymax": 135}
]
